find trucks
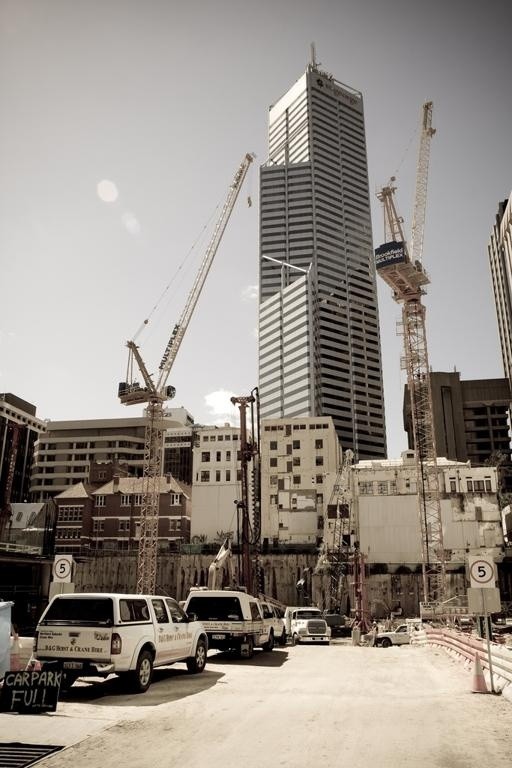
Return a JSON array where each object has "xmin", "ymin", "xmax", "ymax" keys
[{"xmin": 281, "ymin": 606, "xmax": 331, "ymax": 645}]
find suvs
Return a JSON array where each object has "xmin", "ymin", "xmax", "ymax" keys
[
  {"xmin": 182, "ymin": 590, "xmax": 286, "ymax": 659},
  {"xmin": 33, "ymin": 593, "xmax": 208, "ymax": 693}
]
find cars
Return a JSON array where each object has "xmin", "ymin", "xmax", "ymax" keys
[{"xmin": 376, "ymin": 624, "xmax": 419, "ymax": 648}]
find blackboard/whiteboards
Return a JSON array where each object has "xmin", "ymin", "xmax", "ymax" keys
[{"xmin": 1, "ymin": 670, "xmax": 62, "ymax": 713}]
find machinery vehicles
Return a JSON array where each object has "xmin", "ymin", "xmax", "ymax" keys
[
  {"xmin": 229, "ymin": 387, "xmax": 261, "ymax": 596},
  {"xmin": 178, "ymin": 536, "xmax": 247, "ymax": 609}
]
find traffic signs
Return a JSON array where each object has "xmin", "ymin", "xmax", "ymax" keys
[
  {"xmin": 469, "ymin": 556, "xmax": 495, "ymax": 588},
  {"xmin": 53, "ymin": 555, "xmax": 72, "ymax": 583}
]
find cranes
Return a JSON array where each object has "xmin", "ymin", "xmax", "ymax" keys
[
  {"xmin": 118, "ymin": 151, "xmax": 258, "ymax": 595},
  {"xmin": 318, "ymin": 449, "xmax": 373, "ymax": 638},
  {"xmin": 374, "ymin": 101, "xmax": 475, "ymax": 634}
]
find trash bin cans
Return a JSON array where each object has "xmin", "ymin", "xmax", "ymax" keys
[{"xmin": 352, "ymin": 625, "xmax": 361, "ymax": 646}]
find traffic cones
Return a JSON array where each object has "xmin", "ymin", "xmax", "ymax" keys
[{"xmin": 470, "ymin": 652, "xmax": 488, "ymax": 693}]
